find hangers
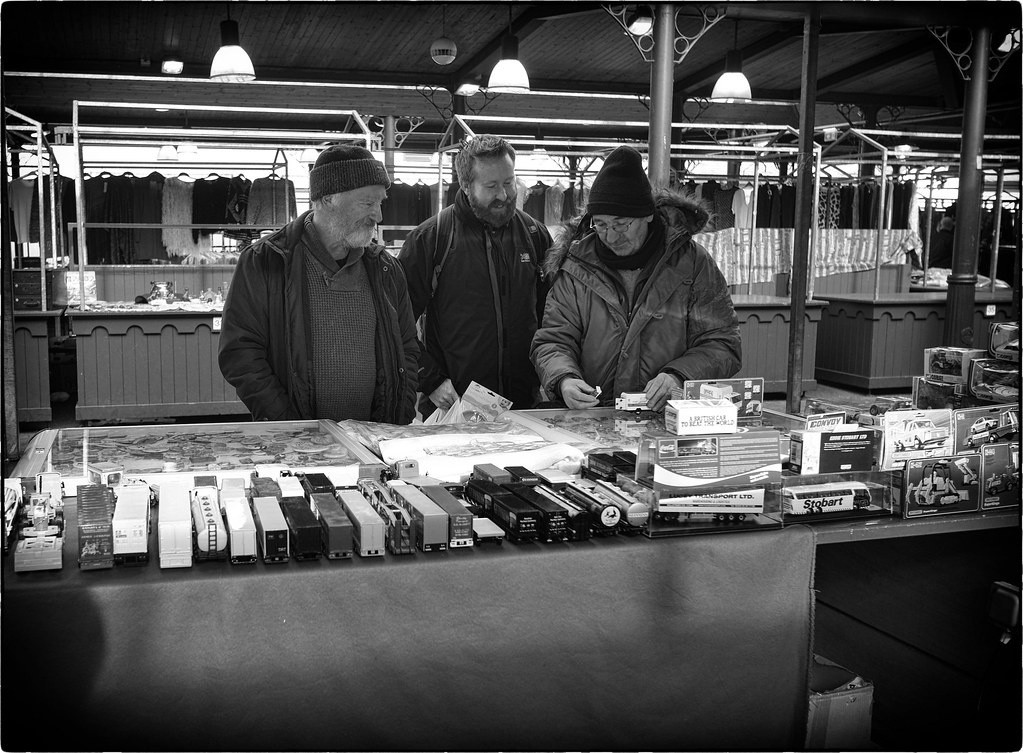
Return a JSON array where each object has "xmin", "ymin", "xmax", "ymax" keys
[
  {"xmin": 389, "ymin": 171, "xmax": 922, "ymax": 191},
  {"xmin": 14, "ymin": 160, "xmax": 284, "ymax": 189}
]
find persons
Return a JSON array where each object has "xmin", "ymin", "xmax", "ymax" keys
[
  {"xmin": 927, "ymin": 216, "xmax": 954, "ymax": 270},
  {"xmin": 217, "ymin": 146, "xmax": 420, "ymax": 426},
  {"xmin": 529, "ymin": 146, "xmax": 743, "ymax": 413},
  {"xmin": 397, "ymin": 135, "xmax": 557, "ymax": 423}
]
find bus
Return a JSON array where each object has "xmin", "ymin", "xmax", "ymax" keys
[
  {"xmin": 653, "ymin": 487, "xmax": 765, "ymax": 523},
  {"xmin": 778, "ymin": 481, "xmax": 873, "ymax": 518}
]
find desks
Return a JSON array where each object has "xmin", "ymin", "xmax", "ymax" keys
[{"xmin": 1, "ymin": 512, "xmax": 1022, "ymax": 753}]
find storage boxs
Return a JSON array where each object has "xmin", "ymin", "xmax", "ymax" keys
[
  {"xmin": 800, "ymin": 651, "xmax": 873, "ymax": 752},
  {"xmin": 628, "ymin": 347, "xmax": 1023, "ymax": 540}
]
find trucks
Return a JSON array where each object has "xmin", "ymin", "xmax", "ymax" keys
[{"xmin": 699, "ymin": 382, "xmax": 744, "ymax": 413}]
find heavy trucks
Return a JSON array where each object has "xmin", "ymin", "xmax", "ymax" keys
[{"xmin": 4, "ymin": 466, "xmax": 659, "ymax": 575}]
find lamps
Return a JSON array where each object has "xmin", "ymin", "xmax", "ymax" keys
[
  {"xmin": 752, "ymin": 122, "xmax": 770, "ymax": 156},
  {"xmin": 710, "ymin": 1, "xmax": 753, "ymax": 102},
  {"xmin": 487, "ymin": 0, "xmax": 531, "ymax": 92},
  {"xmin": 209, "ymin": 1, "xmax": 255, "ymax": 83},
  {"xmin": 624, "ymin": 0, "xmax": 653, "ymax": 37},
  {"xmin": 430, "ymin": 0, "xmax": 457, "ymax": 66}
]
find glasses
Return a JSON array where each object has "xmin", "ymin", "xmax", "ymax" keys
[{"xmin": 590, "ymin": 216, "xmax": 636, "ymax": 234}]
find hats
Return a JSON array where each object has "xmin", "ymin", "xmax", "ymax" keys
[
  {"xmin": 587, "ymin": 145, "xmax": 654, "ymax": 219},
  {"xmin": 310, "ymin": 144, "xmax": 391, "ymax": 202}
]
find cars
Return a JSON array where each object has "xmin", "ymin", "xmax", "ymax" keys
[
  {"xmin": 894, "ymin": 419, "xmax": 950, "ymax": 454},
  {"xmin": 971, "ymin": 416, "xmax": 999, "ymax": 436}
]
